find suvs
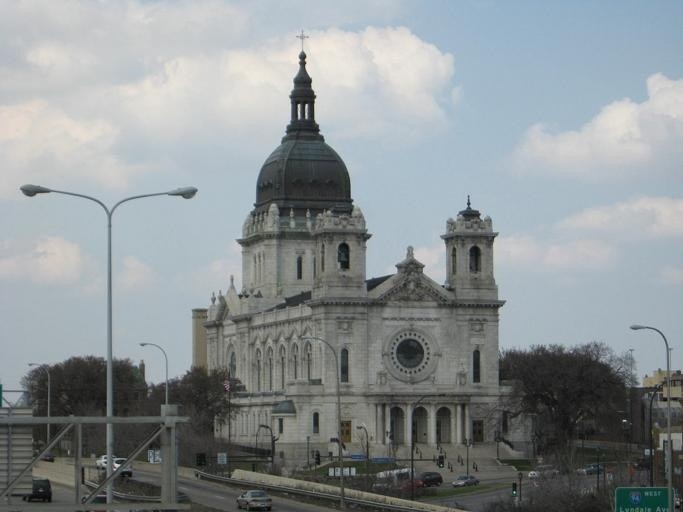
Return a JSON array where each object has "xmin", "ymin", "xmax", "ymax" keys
[{"xmin": 23, "ymin": 477, "xmax": 53, "ymax": 503}]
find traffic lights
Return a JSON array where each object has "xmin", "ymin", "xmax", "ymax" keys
[{"xmin": 512, "ymin": 483, "xmax": 517, "ymax": 497}]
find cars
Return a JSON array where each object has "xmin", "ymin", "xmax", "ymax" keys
[
  {"xmin": 94, "ymin": 452, "xmax": 134, "ymax": 478},
  {"xmin": 236, "ymin": 489, "xmax": 273, "ymax": 512},
  {"xmin": 414, "ymin": 471, "xmax": 444, "ymax": 488},
  {"xmin": 450, "ymin": 474, "xmax": 479, "ymax": 487}
]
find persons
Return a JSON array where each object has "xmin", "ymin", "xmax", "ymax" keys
[{"xmin": 315, "ymin": 451, "xmax": 320, "ymax": 465}]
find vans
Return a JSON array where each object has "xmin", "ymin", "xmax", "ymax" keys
[{"xmin": 372, "ymin": 466, "xmax": 413, "ymax": 493}]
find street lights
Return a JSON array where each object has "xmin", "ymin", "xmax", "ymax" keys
[{"xmin": 627, "ymin": 320, "xmax": 683, "ymax": 512}]
